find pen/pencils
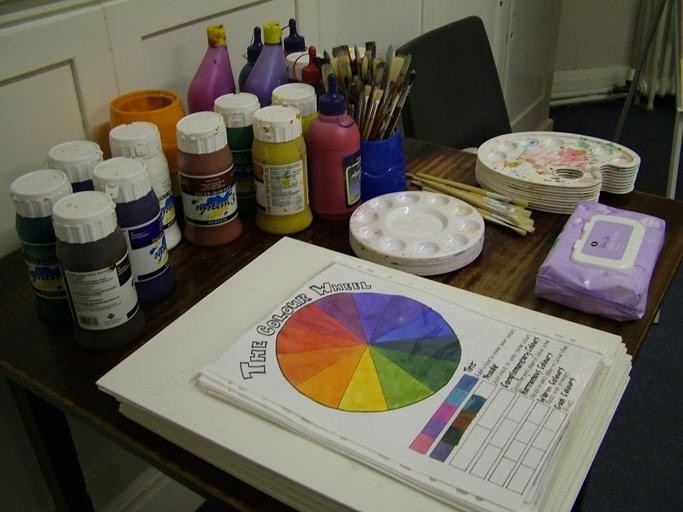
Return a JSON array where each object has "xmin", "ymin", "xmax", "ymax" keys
[{"xmin": 313, "ymin": 42, "xmax": 413, "ymax": 140}]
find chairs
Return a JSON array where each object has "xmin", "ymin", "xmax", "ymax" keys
[{"xmin": 393, "ymin": 16, "xmax": 513, "ymax": 153}]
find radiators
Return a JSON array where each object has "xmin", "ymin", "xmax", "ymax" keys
[{"xmin": 628, "ymin": 0, "xmax": 683, "ymax": 114}]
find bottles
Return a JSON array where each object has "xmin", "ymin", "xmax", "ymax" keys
[
  {"xmin": 8, "ymin": 119, "xmax": 182, "ymax": 353},
  {"xmin": 175, "ymin": 19, "xmax": 360, "ymax": 249}
]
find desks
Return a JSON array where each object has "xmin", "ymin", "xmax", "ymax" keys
[{"xmin": 1, "ymin": 132, "xmax": 682, "ymax": 512}]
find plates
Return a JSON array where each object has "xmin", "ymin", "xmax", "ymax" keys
[
  {"xmin": 348, "ymin": 190, "xmax": 486, "ymax": 277},
  {"xmin": 472, "ymin": 128, "xmax": 641, "ymax": 214}
]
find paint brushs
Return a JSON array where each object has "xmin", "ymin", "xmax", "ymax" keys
[{"xmin": 406, "ymin": 173, "xmax": 536, "ymax": 236}]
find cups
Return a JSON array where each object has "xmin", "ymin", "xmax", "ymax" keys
[
  {"xmin": 361, "ymin": 126, "xmax": 405, "ymax": 202},
  {"xmin": 109, "ymin": 87, "xmax": 186, "ymax": 182}
]
